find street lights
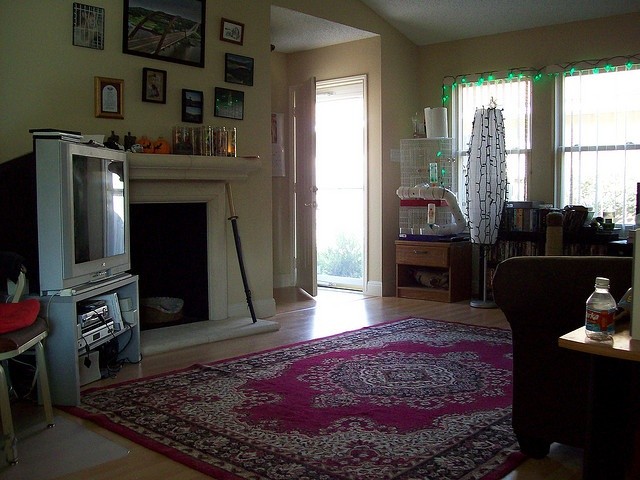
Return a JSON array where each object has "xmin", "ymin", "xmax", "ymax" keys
[{"xmin": 409, "ymin": 268, "xmax": 438, "ymax": 286}]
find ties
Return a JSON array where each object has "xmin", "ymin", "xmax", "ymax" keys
[{"xmin": 0, "ymin": 127, "xmax": 132, "ymax": 296}]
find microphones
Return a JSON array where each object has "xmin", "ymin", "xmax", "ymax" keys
[{"xmin": 0, "ymin": 316, "xmax": 55, "ymax": 466}]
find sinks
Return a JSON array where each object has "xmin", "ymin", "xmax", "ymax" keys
[{"xmin": 493, "ymin": 257, "xmax": 639, "ymax": 460}]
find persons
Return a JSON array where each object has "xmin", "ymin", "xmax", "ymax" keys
[{"xmin": 149, "ymin": 84, "xmax": 160, "ymax": 97}]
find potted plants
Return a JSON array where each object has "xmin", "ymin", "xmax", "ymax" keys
[
  {"xmin": 94, "ymin": 75, "xmax": 125, "ymax": 119},
  {"xmin": 71, "ymin": 4, "xmax": 107, "ymax": 51},
  {"xmin": 181, "ymin": 89, "xmax": 205, "ymax": 125},
  {"xmin": 219, "ymin": 19, "xmax": 249, "ymax": 46},
  {"xmin": 142, "ymin": 68, "xmax": 168, "ymax": 102},
  {"xmin": 124, "ymin": 0, "xmax": 208, "ymax": 73},
  {"xmin": 211, "ymin": 87, "xmax": 247, "ymax": 121},
  {"xmin": 222, "ymin": 54, "xmax": 256, "ymax": 86}
]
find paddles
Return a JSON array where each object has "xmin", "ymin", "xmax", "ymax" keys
[{"xmin": 58, "ymin": 316, "xmax": 525, "ymax": 476}]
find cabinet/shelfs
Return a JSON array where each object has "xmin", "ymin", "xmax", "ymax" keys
[{"xmin": 584, "ymin": 276, "xmax": 617, "ymax": 341}]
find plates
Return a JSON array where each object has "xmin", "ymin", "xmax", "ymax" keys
[
  {"xmin": 494, "ymin": 206, "xmax": 620, "ymax": 267},
  {"xmin": 394, "ymin": 240, "xmax": 473, "ymax": 304}
]
